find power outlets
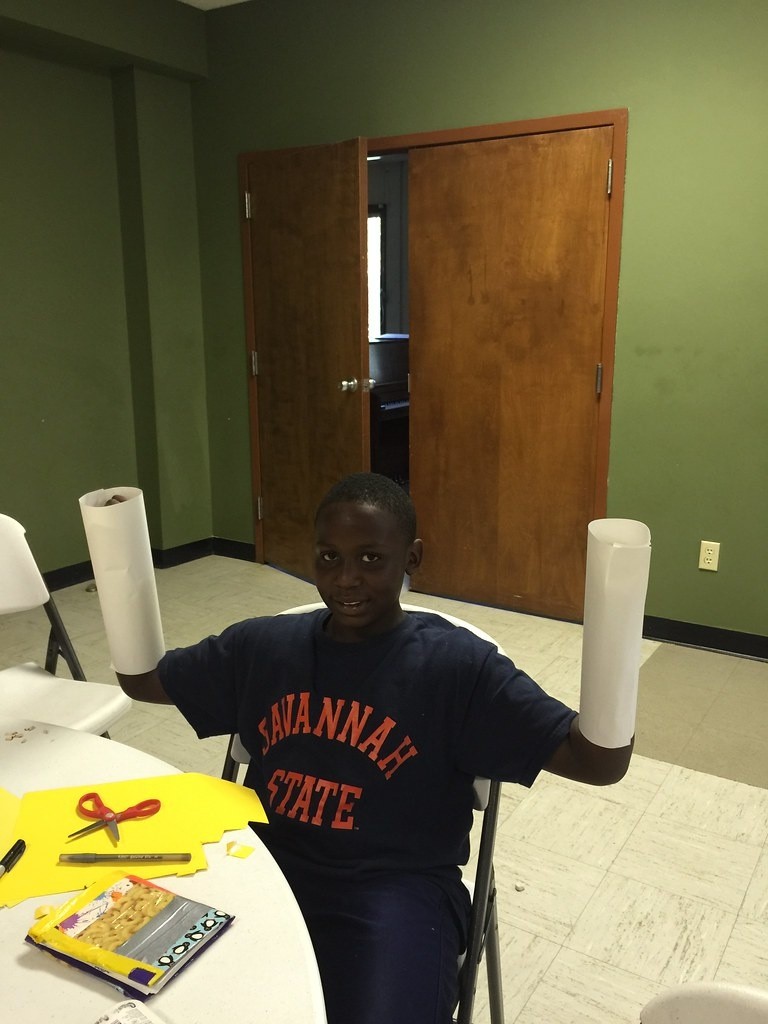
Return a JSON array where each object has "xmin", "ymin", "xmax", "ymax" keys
[{"xmin": 696, "ymin": 538, "xmax": 720, "ymax": 575}]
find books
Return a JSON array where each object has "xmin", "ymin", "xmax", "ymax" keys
[{"xmin": 25, "ymin": 868, "xmax": 237, "ymax": 1003}]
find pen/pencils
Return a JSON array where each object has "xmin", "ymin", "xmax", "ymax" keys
[
  {"xmin": 0, "ymin": 839, "xmax": 26, "ymax": 879},
  {"xmin": 58, "ymin": 852, "xmax": 191, "ymax": 863}
]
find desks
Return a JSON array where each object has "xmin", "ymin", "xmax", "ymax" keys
[{"xmin": 0, "ymin": 715, "xmax": 331, "ymax": 1023}]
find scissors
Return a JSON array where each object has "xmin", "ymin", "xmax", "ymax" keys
[{"xmin": 70, "ymin": 792, "xmax": 161, "ymax": 842}]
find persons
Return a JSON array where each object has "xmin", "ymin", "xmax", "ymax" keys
[{"xmin": 77, "ymin": 473, "xmax": 651, "ymax": 1024}]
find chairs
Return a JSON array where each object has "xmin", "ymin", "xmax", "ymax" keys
[
  {"xmin": 206, "ymin": 602, "xmax": 506, "ymax": 1024},
  {"xmin": 0, "ymin": 511, "xmax": 134, "ymax": 758}
]
